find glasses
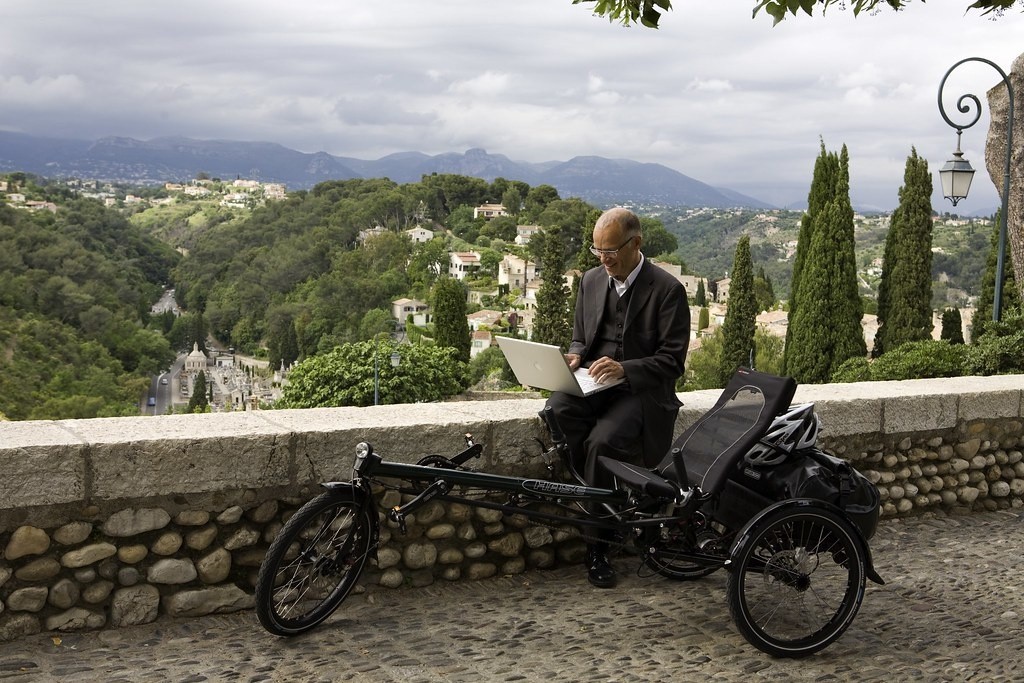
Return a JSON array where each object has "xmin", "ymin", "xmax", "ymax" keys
[{"xmin": 589, "ymin": 237, "xmax": 633, "ymax": 257}]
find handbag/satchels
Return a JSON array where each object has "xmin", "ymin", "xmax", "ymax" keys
[{"xmin": 765, "ymin": 445, "xmax": 881, "ymax": 540}]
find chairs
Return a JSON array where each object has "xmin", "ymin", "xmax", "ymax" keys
[{"xmin": 595, "ymin": 367, "xmax": 799, "ymax": 501}]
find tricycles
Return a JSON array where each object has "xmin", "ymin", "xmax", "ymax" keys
[{"xmin": 254, "ymin": 364, "xmax": 888, "ymax": 660}]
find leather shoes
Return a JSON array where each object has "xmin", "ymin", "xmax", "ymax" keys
[{"xmin": 584, "ymin": 550, "xmax": 616, "ymax": 587}]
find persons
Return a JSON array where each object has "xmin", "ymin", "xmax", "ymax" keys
[{"xmin": 538, "ymin": 208, "xmax": 692, "ymax": 589}]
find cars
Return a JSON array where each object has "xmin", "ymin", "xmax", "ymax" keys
[
  {"xmin": 161, "ymin": 378, "xmax": 169, "ymax": 385},
  {"xmin": 147, "ymin": 397, "xmax": 157, "ymax": 407}
]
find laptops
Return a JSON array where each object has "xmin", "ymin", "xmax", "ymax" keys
[{"xmin": 495, "ymin": 335, "xmax": 626, "ymax": 397}]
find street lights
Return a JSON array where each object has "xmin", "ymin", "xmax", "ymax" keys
[{"xmin": 937, "ymin": 56, "xmax": 1016, "ymax": 321}]
